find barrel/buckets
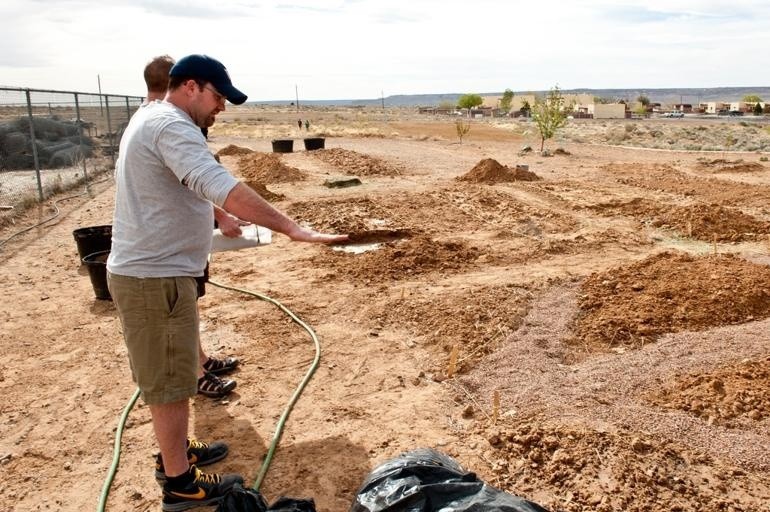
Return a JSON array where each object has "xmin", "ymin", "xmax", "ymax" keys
[
  {"xmin": 304, "ymin": 138, "xmax": 325, "ymax": 149},
  {"xmin": 272, "ymin": 139, "xmax": 293, "ymax": 152},
  {"xmin": 82, "ymin": 251, "xmax": 114, "ymax": 301},
  {"xmin": 73, "ymin": 225, "xmax": 113, "ymax": 260}
]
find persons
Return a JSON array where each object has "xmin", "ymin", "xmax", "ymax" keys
[
  {"xmin": 106, "ymin": 53, "xmax": 350, "ymax": 512},
  {"xmin": 141, "ymin": 54, "xmax": 252, "ymax": 398}
]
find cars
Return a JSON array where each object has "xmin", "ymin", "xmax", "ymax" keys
[{"xmin": 667, "ymin": 111, "xmax": 684, "ymax": 118}]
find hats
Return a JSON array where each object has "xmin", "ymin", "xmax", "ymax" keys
[{"xmin": 168, "ymin": 54, "xmax": 248, "ymax": 107}]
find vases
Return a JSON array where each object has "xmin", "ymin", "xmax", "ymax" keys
[
  {"xmin": 72, "ymin": 225, "xmax": 112, "ymax": 301},
  {"xmin": 272, "ymin": 139, "xmax": 324, "ymax": 153}
]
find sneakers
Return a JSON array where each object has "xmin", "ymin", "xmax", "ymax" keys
[
  {"xmin": 153, "ymin": 438, "xmax": 229, "ymax": 481},
  {"xmin": 196, "ymin": 374, "xmax": 237, "ymax": 397},
  {"xmin": 161, "ymin": 463, "xmax": 244, "ymax": 512},
  {"xmin": 202, "ymin": 357, "xmax": 241, "ymax": 374}
]
